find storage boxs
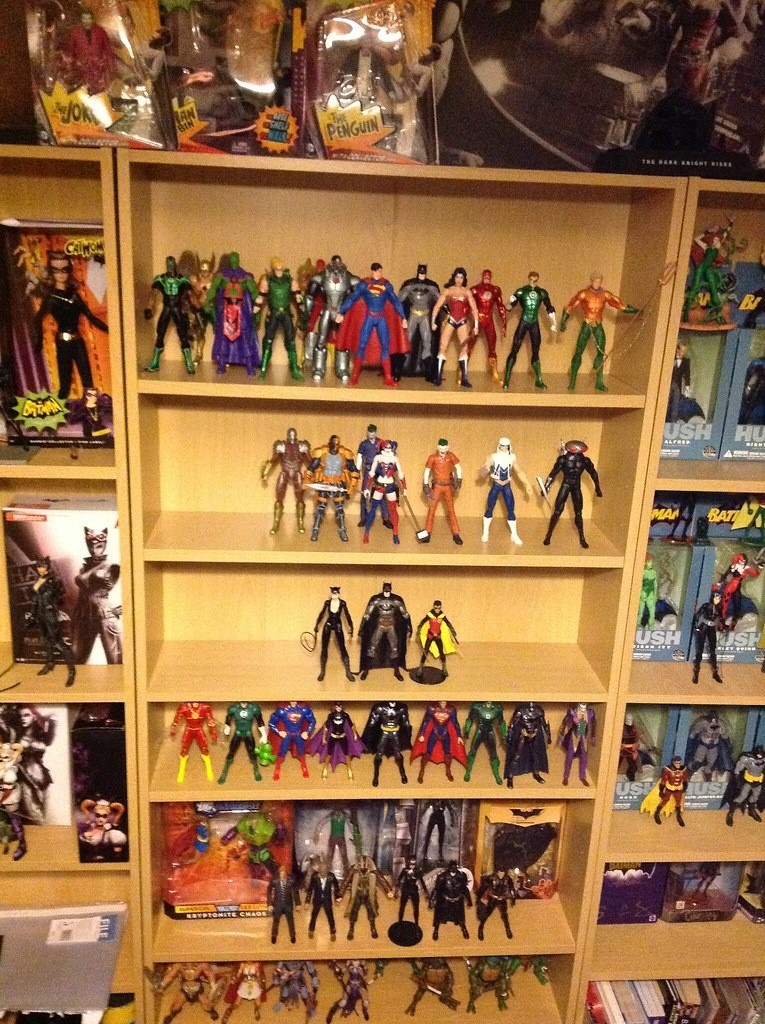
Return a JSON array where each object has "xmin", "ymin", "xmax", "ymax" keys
[
  {"xmin": 23, "ymin": 0, "xmax": 439, "ymax": 164},
  {"xmin": 464, "ymin": 800, "xmax": 568, "ymax": 899},
  {"xmin": 660, "ymin": 327, "xmax": 765, "ymax": 463},
  {"xmin": 597, "ymin": 861, "xmax": 765, "ymax": 926},
  {"xmin": 613, "ymin": 705, "xmax": 765, "ymax": 811},
  {"xmin": 631, "ymin": 500, "xmax": 765, "ymax": 663},
  {"xmin": 2, "ymin": 494, "xmax": 120, "ymax": 666}
]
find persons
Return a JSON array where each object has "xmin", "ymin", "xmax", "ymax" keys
[
  {"xmin": 622, "ymin": 711, "xmax": 765, "ymax": 826},
  {"xmin": 216, "ymin": 803, "xmax": 556, "ymax": 941},
  {"xmin": 477, "ymin": 438, "xmax": 524, "ymax": 545},
  {"xmin": 633, "ymin": 553, "xmax": 660, "ymax": 632},
  {"xmin": 540, "ymin": 439, "xmax": 603, "ymax": 549},
  {"xmin": 0, "ymin": 740, "xmax": 28, "ymax": 860},
  {"xmin": 169, "ymin": 702, "xmax": 596, "ymax": 789},
  {"xmin": 151, "ymin": 955, "xmax": 551, "ymax": 1023},
  {"xmin": 418, "ymin": 439, "xmax": 466, "ymax": 545},
  {"xmin": 20, "ymin": 557, "xmax": 76, "ymax": 686},
  {"xmin": 261, "ymin": 424, "xmax": 408, "ymax": 543},
  {"xmin": 141, "ymin": 251, "xmax": 641, "ymax": 393},
  {"xmin": 687, "ymin": 589, "xmax": 723, "ymax": 685},
  {"xmin": 60, "ymin": 10, "xmax": 115, "ymax": 95},
  {"xmin": 32, "ymin": 246, "xmax": 109, "ymax": 401},
  {"xmin": 338, "ymin": 30, "xmax": 395, "ymax": 103},
  {"xmin": 310, "ymin": 581, "xmax": 462, "ymax": 682},
  {"xmin": 664, "ymin": 341, "xmax": 690, "ymax": 423},
  {"xmin": 683, "ymin": 226, "xmax": 733, "ymax": 325},
  {"xmin": 714, "ymin": 550, "xmax": 756, "ymax": 632}
]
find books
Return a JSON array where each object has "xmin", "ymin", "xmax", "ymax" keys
[{"xmin": 583, "ymin": 977, "xmax": 765, "ymax": 1024}]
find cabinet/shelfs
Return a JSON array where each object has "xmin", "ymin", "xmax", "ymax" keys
[{"xmin": 0, "ymin": 144, "xmax": 765, "ymax": 1024}]
